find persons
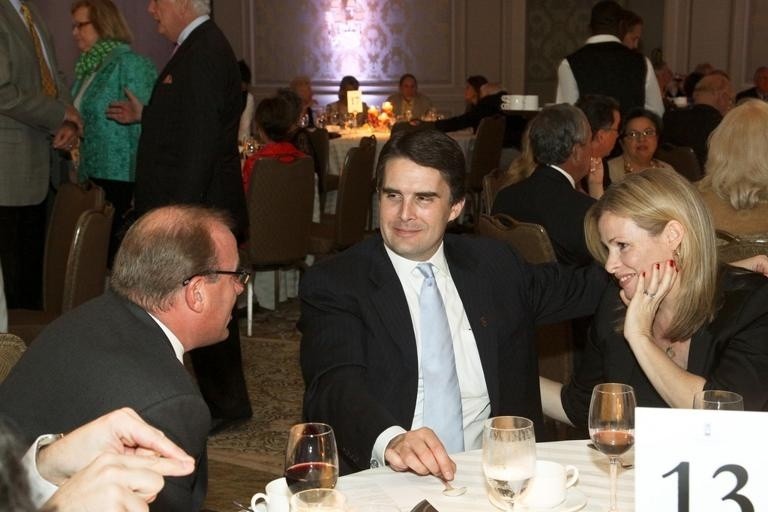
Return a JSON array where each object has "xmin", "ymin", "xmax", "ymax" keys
[
  {"xmin": 0, "ymin": 406, "xmax": 195, "ymax": 511},
  {"xmin": 296, "ymin": 127, "xmax": 766, "ymax": 485},
  {"xmin": 64, "ymin": 0, "xmax": 158, "ymax": 263},
  {"xmin": 239, "ymin": 73, "xmax": 505, "ymax": 189},
  {"xmin": 2, "ymin": 202, "xmax": 244, "ymax": 510},
  {"xmin": 0, "ymin": 0, "xmax": 82, "ymax": 310},
  {"xmin": 106, "ymin": 0, "xmax": 250, "ymax": 435},
  {"xmin": 537, "ymin": 167, "xmax": 766, "ymax": 436}
]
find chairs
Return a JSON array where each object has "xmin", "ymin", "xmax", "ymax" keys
[{"xmin": 1, "ymin": 113, "xmax": 767, "ymax": 440}]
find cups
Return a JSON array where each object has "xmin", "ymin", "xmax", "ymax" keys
[
  {"xmin": 501, "ymin": 95, "xmax": 539, "ymax": 110},
  {"xmin": 693, "ymin": 390, "xmax": 744, "ymax": 411},
  {"xmin": 672, "ymin": 97, "xmax": 687, "ymax": 106},
  {"xmin": 526, "ymin": 460, "xmax": 578, "ymax": 510},
  {"xmin": 250, "ymin": 477, "xmax": 287, "ymax": 511},
  {"xmin": 288, "ymin": 487, "xmax": 346, "ymax": 511}
]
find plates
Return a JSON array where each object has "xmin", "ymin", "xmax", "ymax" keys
[{"xmin": 521, "ymin": 489, "xmax": 592, "ymax": 512}]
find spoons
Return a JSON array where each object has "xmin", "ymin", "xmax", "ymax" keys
[
  {"xmin": 433, "ymin": 474, "xmax": 466, "ymax": 496},
  {"xmin": 587, "ymin": 443, "xmax": 633, "ymax": 470}
]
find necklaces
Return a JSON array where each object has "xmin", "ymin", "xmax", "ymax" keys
[{"xmin": 655, "ymin": 327, "xmax": 692, "ymax": 360}]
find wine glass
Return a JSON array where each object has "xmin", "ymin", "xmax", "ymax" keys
[
  {"xmin": 284, "ymin": 421, "xmax": 352, "ymax": 511},
  {"xmin": 295, "ymin": 101, "xmax": 458, "ymax": 138},
  {"xmin": 481, "ymin": 413, "xmax": 537, "ymax": 512},
  {"xmin": 243, "ymin": 138, "xmax": 258, "ymax": 160},
  {"xmin": 586, "ymin": 382, "xmax": 637, "ymax": 512}
]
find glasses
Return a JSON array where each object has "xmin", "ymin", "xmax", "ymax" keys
[
  {"xmin": 181, "ymin": 268, "xmax": 252, "ymax": 288},
  {"xmin": 621, "ymin": 128, "xmax": 657, "ymax": 140}
]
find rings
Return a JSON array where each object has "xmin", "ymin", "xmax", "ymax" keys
[
  {"xmin": 68, "ymin": 143, "xmax": 73, "ymax": 151},
  {"xmin": 644, "ymin": 290, "xmax": 656, "ymax": 299}
]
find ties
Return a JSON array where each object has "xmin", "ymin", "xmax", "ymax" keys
[
  {"xmin": 169, "ymin": 44, "xmax": 180, "ymax": 60},
  {"xmin": 413, "ymin": 262, "xmax": 466, "ymax": 455},
  {"xmin": 20, "ymin": 3, "xmax": 59, "ymax": 99}
]
